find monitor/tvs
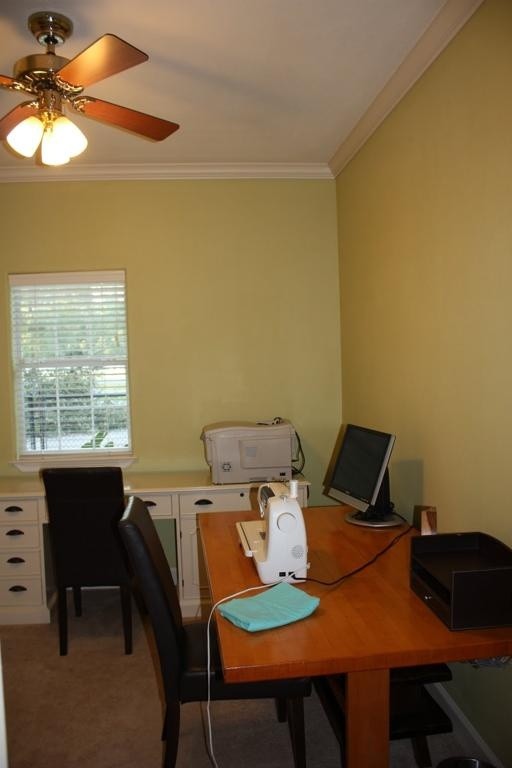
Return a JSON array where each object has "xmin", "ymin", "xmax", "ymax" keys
[{"xmin": 321, "ymin": 423, "xmax": 403, "ymax": 529}]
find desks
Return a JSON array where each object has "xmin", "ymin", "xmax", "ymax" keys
[
  {"xmin": 193, "ymin": 507, "xmax": 510, "ymax": 767},
  {"xmin": 2, "ymin": 469, "xmax": 312, "ymax": 629}
]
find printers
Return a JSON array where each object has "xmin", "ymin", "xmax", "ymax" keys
[{"xmin": 200, "ymin": 417, "xmax": 292, "ymax": 486}]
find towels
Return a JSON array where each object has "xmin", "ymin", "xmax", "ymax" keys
[{"xmin": 215, "ymin": 584, "xmax": 323, "ymax": 634}]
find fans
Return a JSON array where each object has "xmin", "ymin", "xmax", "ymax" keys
[{"xmin": 0, "ymin": 11, "xmax": 180, "ymax": 146}]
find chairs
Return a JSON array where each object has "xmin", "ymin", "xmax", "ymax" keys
[
  {"xmin": 119, "ymin": 496, "xmax": 315, "ymax": 766},
  {"xmin": 40, "ymin": 467, "xmax": 138, "ymax": 657}
]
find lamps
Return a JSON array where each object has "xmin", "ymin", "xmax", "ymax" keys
[{"xmin": 4, "ymin": 108, "xmax": 88, "ymax": 167}]
